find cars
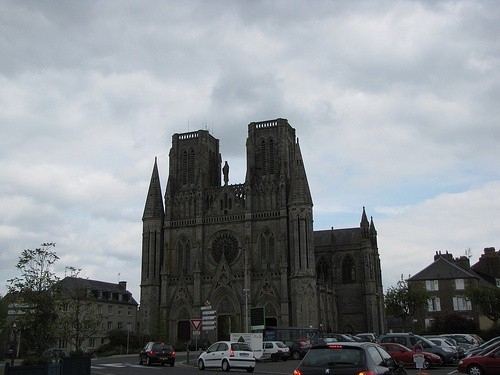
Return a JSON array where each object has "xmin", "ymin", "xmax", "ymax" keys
[
  {"xmin": 283, "ymin": 339, "xmax": 311, "ymax": 360},
  {"xmin": 293, "ymin": 340, "xmax": 408, "ymax": 375},
  {"xmin": 262, "ymin": 341, "xmax": 289, "ymax": 362},
  {"xmin": 378, "ymin": 331, "xmax": 484, "ymax": 364},
  {"xmin": 379, "ymin": 343, "xmax": 442, "ymax": 370},
  {"xmin": 309, "ymin": 333, "xmax": 380, "ymax": 346},
  {"xmin": 456, "ymin": 337, "xmax": 500, "ymax": 375},
  {"xmin": 137, "ymin": 341, "xmax": 176, "ymax": 366},
  {"xmin": 196, "ymin": 341, "xmax": 256, "ymax": 373}
]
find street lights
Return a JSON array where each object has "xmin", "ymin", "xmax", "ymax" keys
[{"xmin": 243, "ymin": 288, "xmax": 250, "ymax": 332}]
